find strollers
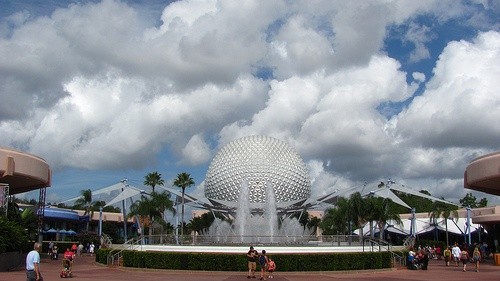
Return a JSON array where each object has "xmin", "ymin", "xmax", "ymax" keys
[{"xmin": 59, "ymin": 258, "xmax": 74, "ymax": 278}]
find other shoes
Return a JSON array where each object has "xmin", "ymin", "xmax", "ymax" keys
[
  {"xmin": 247, "ymin": 276, "xmax": 250, "ymax": 279},
  {"xmin": 260, "ymin": 277, "xmax": 263, "ymax": 280},
  {"xmin": 253, "ymin": 276, "xmax": 256, "ymax": 278}
]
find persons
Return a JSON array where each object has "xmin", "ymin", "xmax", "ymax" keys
[
  {"xmin": 71, "ymin": 243, "xmax": 77, "ymax": 256},
  {"xmin": 85, "ymin": 242, "xmax": 95, "ymax": 256},
  {"xmin": 26, "ymin": 242, "xmax": 43, "ymax": 281},
  {"xmin": 267, "ymin": 258, "xmax": 276, "ymax": 278},
  {"xmin": 247, "ymin": 246, "xmax": 259, "ymax": 278},
  {"xmin": 407, "ymin": 245, "xmax": 441, "ymax": 270},
  {"xmin": 64, "ymin": 248, "xmax": 74, "ymax": 268},
  {"xmin": 259, "ymin": 250, "xmax": 268, "ymax": 281},
  {"xmin": 78, "ymin": 243, "xmax": 84, "ymax": 255},
  {"xmin": 48, "ymin": 243, "xmax": 59, "ymax": 260},
  {"xmin": 443, "ymin": 241, "xmax": 490, "ymax": 272}
]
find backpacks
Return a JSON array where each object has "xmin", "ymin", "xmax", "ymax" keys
[
  {"xmin": 461, "ymin": 252, "xmax": 467, "ymax": 259},
  {"xmin": 260, "ymin": 256, "xmax": 265, "ymax": 264},
  {"xmin": 474, "ymin": 251, "xmax": 479, "ymax": 260}
]
